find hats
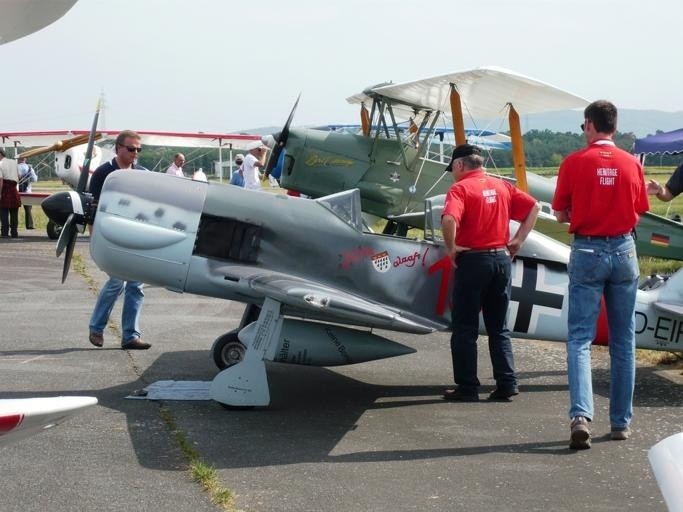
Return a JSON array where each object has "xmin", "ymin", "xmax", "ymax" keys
[
  {"xmin": 443, "ymin": 144, "xmax": 483, "ymax": 172},
  {"xmin": 235, "ymin": 153, "xmax": 245, "ymax": 163},
  {"xmin": 245, "ymin": 139, "xmax": 263, "ymax": 151}
]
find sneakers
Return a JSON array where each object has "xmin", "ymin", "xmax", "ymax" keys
[
  {"xmin": 444, "ymin": 382, "xmax": 481, "ymax": 402},
  {"xmin": 121, "ymin": 336, "xmax": 152, "ymax": 349},
  {"xmin": 568, "ymin": 414, "xmax": 592, "ymax": 449},
  {"xmin": 490, "ymin": 386, "xmax": 520, "ymax": 400},
  {"xmin": 89, "ymin": 325, "xmax": 103, "ymax": 347},
  {"xmin": 0, "ymin": 229, "xmax": 19, "ymax": 239},
  {"xmin": 610, "ymin": 417, "xmax": 632, "ymax": 440}
]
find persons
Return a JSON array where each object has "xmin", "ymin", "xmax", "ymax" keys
[
  {"xmin": 87, "ymin": 131, "xmax": 152, "ymax": 350},
  {"xmin": 551, "ymin": 99, "xmax": 650, "ymax": 451},
  {"xmin": 165, "ymin": 152, "xmax": 185, "ymax": 177},
  {"xmin": 440, "ymin": 145, "xmax": 542, "ymax": 401},
  {"xmin": 0, "ymin": 149, "xmax": 21, "ymax": 238},
  {"xmin": 16, "ymin": 157, "xmax": 38, "ymax": 229},
  {"xmin": 238, "ymin": 142, "xmax": 268, "ymax": 191},
  {"xmin": 229, "ymin": 153, "xmax": 245, "ymax": 187},
  {"xmin": 646, "ymin": 162, "xmax": 683, "ymax": 202}
]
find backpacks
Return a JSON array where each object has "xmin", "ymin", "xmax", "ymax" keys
[{"xmin": 19, "ymin": 164, "xmax": 31, "ymax": 192}]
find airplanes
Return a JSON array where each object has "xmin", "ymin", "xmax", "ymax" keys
[
  {"xmin": 37, "ymin": 91, "xmax": 681, "ymax": 413},
  {"xmin": 0, "ymin": 130, "xmax": 149, "ymax": 241},
  {"xmin": 0, "ymin": 392, "xmax": 100, "ymax": 454},
  {"xmin": 241, "ymin": 63, "xmax": 681, "ymax": 264}
]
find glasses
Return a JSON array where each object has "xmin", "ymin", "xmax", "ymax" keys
[
  {"xmin": 117, "ymin": 141, "xmax": 143, "ymax": 154},
  {"xmin": 580, "ymin": 119, "xmax": 591, "ymax": 132}
]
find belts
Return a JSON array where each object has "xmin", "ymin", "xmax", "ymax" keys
[
  {"xmin": 460, "ymin": 244, "xmax": 507, "ymax": 255},
  {"xmin": 573, "ymin": 231, "xmax": 632, "ymax": 241}
]
